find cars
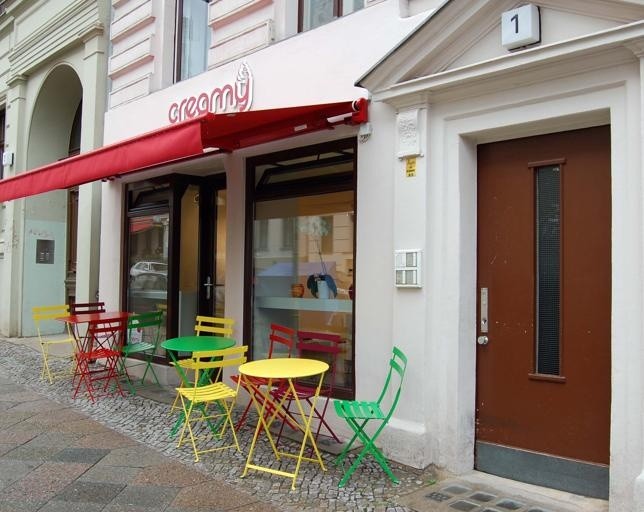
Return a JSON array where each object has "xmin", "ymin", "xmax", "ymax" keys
[{"xmin": 130, "ymin": 261, "xmax": 167, "ymax": 302}]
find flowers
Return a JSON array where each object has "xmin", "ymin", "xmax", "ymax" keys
[{"xmin": 298, "ymin": 215, "xmax": 341, "ymax": 298}]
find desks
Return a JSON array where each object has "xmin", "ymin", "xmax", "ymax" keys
[{"xmin": 161, "ymin": 335, "xmax": 236, "ymax": 441}]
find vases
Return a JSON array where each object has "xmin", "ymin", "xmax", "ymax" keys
[{"xmin": 318, "ymin": 280, "xmax": 329, "ymax": 301}]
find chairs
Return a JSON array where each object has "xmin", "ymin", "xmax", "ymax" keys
[{"xmin": 32, "ymin": 302, "xmax": 162, "ymax": 403}]
